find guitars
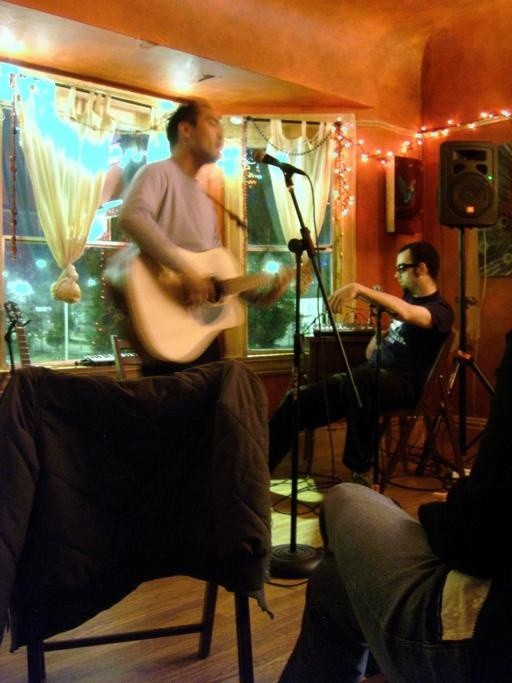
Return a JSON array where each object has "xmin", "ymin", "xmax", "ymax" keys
[
  {"xmin": 5, "ymin": 301, "xmax": 31, "ymax": 369},
  {"xmin": 128, "ymin": 247, "xmax": 312, "ymax": 363}
]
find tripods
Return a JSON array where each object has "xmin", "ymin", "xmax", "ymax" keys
[{"xmin": 416, "ymin": 229, "xmax": 495, "ymax": 475}]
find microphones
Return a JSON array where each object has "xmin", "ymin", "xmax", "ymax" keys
[{"xmin": 252, "ymin": 148, "xmax": 305, "ymax": 175}]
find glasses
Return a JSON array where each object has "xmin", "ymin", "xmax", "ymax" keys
[{"xmin": 395, "ymin": 263, "xmax": 420, "ymax": 273}]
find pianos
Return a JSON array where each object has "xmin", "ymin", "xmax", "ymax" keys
[{"xmin": 83, "ymin": 353, "xmax": 136, "ymax": 367}]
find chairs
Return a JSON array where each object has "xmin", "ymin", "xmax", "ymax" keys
[
  {"xmin": 1, "ymin": 356, "xmax": 273, "ymax": 682},
  {"xmin": 371, "ymin": 331, "xmax": 467, "ymax": 496}
]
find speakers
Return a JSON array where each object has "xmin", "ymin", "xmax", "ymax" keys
[{"xmin": 438, "ymin": 140, "xmax": 512, "ymax": 228}]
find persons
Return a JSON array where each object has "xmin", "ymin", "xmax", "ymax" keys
[
  {"xmin": 120, "ymin": 103, "xmax": 296, "ymax": 377},
  {"xmin": 269, "ymin": 242, "xmax": 453, "ymax": 488},
  {"xmin": 277, "ymin": 329, "xmax": 512, "ymax": 681}
]
